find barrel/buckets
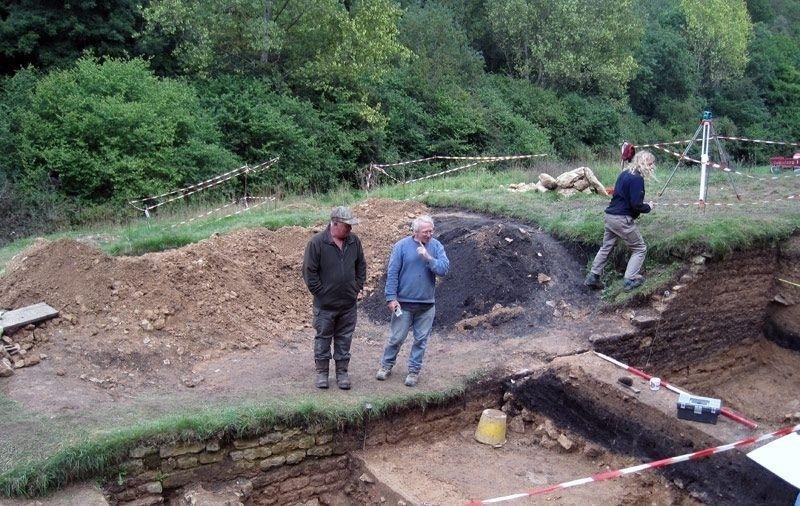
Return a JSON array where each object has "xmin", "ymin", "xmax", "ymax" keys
[{"xmin": 472, "ymin": 408, "xmax": 508, "ymax": 448}]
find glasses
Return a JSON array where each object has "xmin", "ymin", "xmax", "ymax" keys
[{"xmin": 417, "ymin": 231, "xmax": 434, "ymax": 235}]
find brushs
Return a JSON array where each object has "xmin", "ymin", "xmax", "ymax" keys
[{"xmin": 618, "ymin": 376, "xmax": 641, "ymax": 393}]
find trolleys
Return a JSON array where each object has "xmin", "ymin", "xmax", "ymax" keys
[{"xmin": 769, "ymin": 156, "xmax": 800, "ymax": 176}]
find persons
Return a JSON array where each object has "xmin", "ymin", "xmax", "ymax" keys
[
  {"xmin": 377, "ymin": 214, "xmax": 450, "ymax": 387},
  {"xmin": 301, "ymin": 205, "xmax": 367, "ymax": 389},
  {"xmin": 582, "ymin": 150, "xmax": 656, "ymax": 293}
]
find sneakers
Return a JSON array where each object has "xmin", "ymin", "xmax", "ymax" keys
[
  {"xmin": 623, "ymin": 275, "xmax": 644, "ymax": 292},
  {"xmin": 583, "ymin": 273, "xmax": 605, "ymax": 289},
  {"xmin": 375, "ymin": 366, "xmax": 392, "ymax": 380},
  {"xmin": 404, "ymin": 371, "xmax": 420, "ymax": 385}
]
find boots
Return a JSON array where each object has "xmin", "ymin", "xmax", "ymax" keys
[
  {"xmin": 315, "ymin": 359, "xmax": 330, "ymax": 388},
  {"xmin": 335, "ymin": 359, "xmax": 352, "ymax": 389}
]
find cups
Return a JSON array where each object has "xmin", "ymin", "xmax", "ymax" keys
[{"xmin": 650, "ymin": 377, "xmax": 661, "ymax": 391}]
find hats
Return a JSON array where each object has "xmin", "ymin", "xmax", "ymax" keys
[{"xmin": 330, "ymin": 206, "xmax": 359, "ymax": 225}]
